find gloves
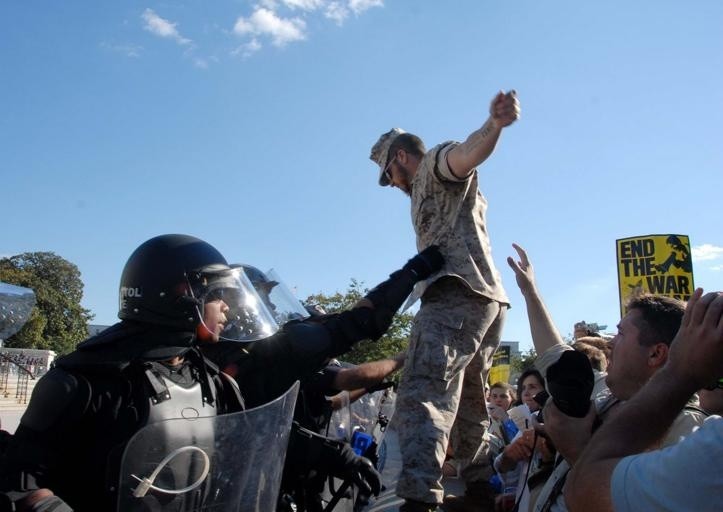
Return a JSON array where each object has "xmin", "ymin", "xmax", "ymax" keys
[
  {"xmin": 332, "ymin": 441, "xmax": 380, "ymax": 499},
  {"xmin": 366, "ymin": 381, "xmax": 395, "ymax": 394},
  {"xmin": 407, "ymin": 245, "xmax": 446, "ymax": 281}
]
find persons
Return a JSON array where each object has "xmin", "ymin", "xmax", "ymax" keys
[
  {"xmin": 2, "ymin": 233, "xmax": 711, "ymax": 510},
  {"xmin": 370, "ymin": 88, "xmax": 520, "ymax": 512}
]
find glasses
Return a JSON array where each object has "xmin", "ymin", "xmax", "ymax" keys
[{"xmin": 384, "ymin": 153, "xmax": 398, "ymax": 180}]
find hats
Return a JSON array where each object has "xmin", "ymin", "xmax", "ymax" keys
[{"xmin": 369, "ymin": 128, "xmax": 407, "ymax": 186}]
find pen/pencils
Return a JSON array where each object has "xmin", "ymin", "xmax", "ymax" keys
[{"xmin": 525, "ymin": 419, "xmax": 528, "ymax": 428}]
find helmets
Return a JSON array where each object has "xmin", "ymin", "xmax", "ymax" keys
[
  {"xmin": 118, "ymin": 233, "xmax": 279, "ymax": 342},
  {"xmin": 228, "ymin": 263, "xmax": 312, "ymax": 328}
]
